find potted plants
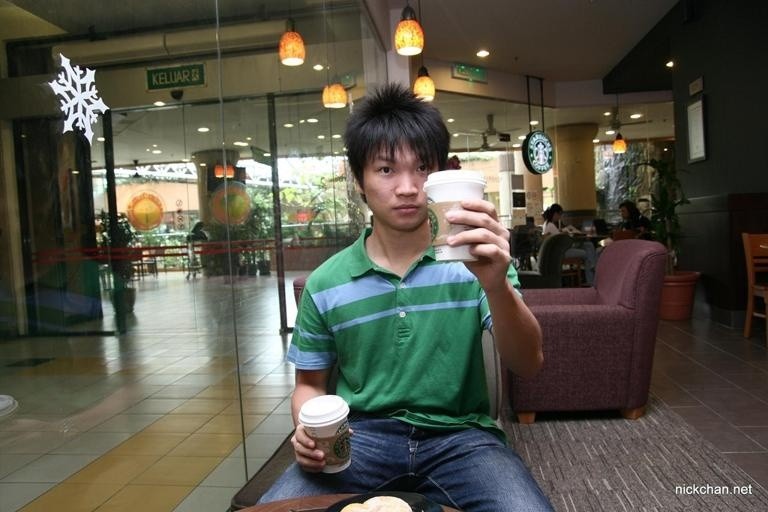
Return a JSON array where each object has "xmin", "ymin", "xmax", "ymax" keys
[
  {"xmin": 227, "ymin": 206, "xmax": 275, "ymax": 275},
  {"xmin": 634, "ymin": 159, "xmax": 701, "ymax": 321}
]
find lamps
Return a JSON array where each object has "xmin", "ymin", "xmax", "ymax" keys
[
  {"xmin": 612, "ymin": 93, "xmax": 626, "ymax": 153},
  {"xmin": 322, "ymin": 0, "xmax": 348, "ymax": 108},
  {"xmin": 214, "ymin": 158, "xmax": 235, "ymax": 179},
  {"xmin": 278, "ymin": 0, "xmax": 305, "ymax": 65},
  {"xmin": 394, "ymin": 0, "xmax": 424, "ymax": 56},
  {"xmin": 413, "ymin": 54, "xmax": 435, "ymax": 101}
]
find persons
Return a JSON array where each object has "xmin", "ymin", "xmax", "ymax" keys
[
  {"xmin": 542, "ymin": 204, "xmax": 586, "ymax": 259},
  {"xmin": 259, "ymin": 79, "xmax": 556, "ymax": 512},
  {"xmin": 619, "ymin": 200, "xmax": 652, "ymax": 240}
]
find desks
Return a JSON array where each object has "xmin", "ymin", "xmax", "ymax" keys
[{"xmin": 232, "ymin": 493, "xmax": 460, "ymax": 512}]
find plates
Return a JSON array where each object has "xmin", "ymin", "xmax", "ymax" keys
[{"xmin": 327, "ymin": 491, "xmax": 446, "ymax": 512}]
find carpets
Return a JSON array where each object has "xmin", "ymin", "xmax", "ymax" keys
[{"xmin": 500, "ymin": 388, "xmax": 768, "ymax": 511}]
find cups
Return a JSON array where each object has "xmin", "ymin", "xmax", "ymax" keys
[
  {"xmin": 422, "ymin": 168, "xmax": 488, "ymax": 264},
  {"xmin": 297, "ymin": 395, "xmax": 352, "ymax": 476},
  {"xmin": 583, "ymin": 226, "xmax": 591, "ymax": 236}
]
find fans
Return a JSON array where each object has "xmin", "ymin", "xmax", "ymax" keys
[
  {"xmin": 464, "ymin": 113, "xmax": 522, "ymax": 137},
  {"xmin": 473, "ymin": 132, "xmax": 498, "ymax": 154}
]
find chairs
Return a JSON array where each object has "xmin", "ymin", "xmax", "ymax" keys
[
  {"xmin": 516, "ymin": 233, "xmax": 571, "ymax": 289},
  {"xmin": 501, "ymin": 239, "xmax": 667, "ymax": 423},
  {"xmin": 742, "ymin": 232, "xmax": 768, "ymax": 345},
  {"xmin": 543, "ymin": 233, "xmax": 581, "ymax": 279}
]
